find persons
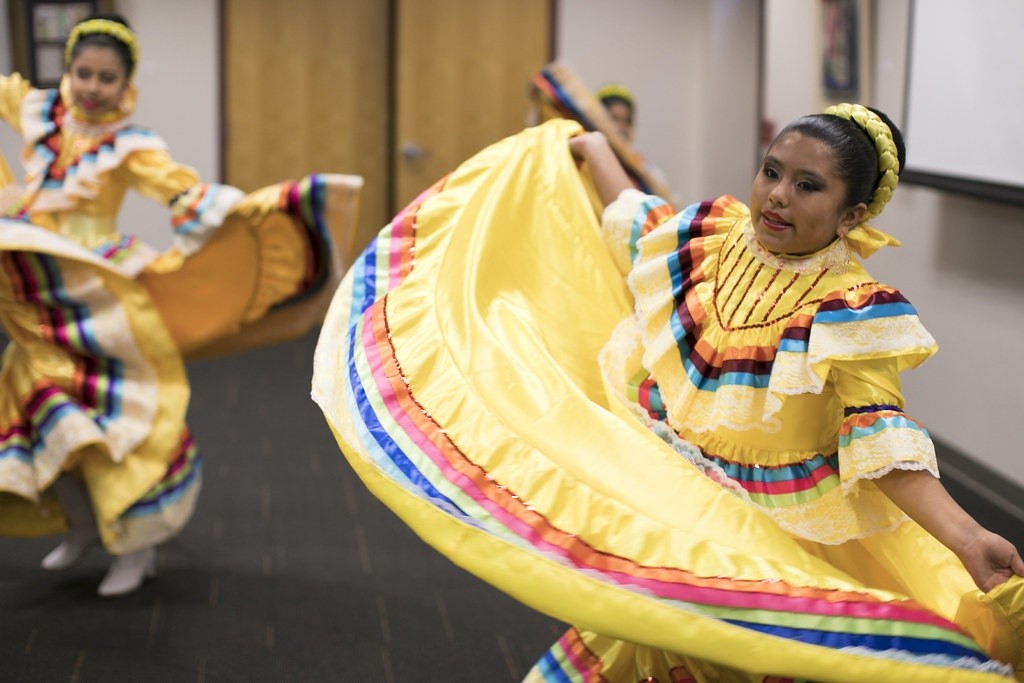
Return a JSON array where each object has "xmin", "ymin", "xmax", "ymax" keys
[
  {"xmin": 568, "ymin": 86, "xmax": 1024, "ymax": 683},
  {"xmin": 0, "ymin": 10, "xmax": 255, "ymax": 597}
]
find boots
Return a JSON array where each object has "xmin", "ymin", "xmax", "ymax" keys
[
  {"xmin": 40, "ymin": 470, "xmax": 97, "ymax": 573},
  {"xmin": 97, "ymin": 548, "xmax": 155, "ymax": 594}
]
